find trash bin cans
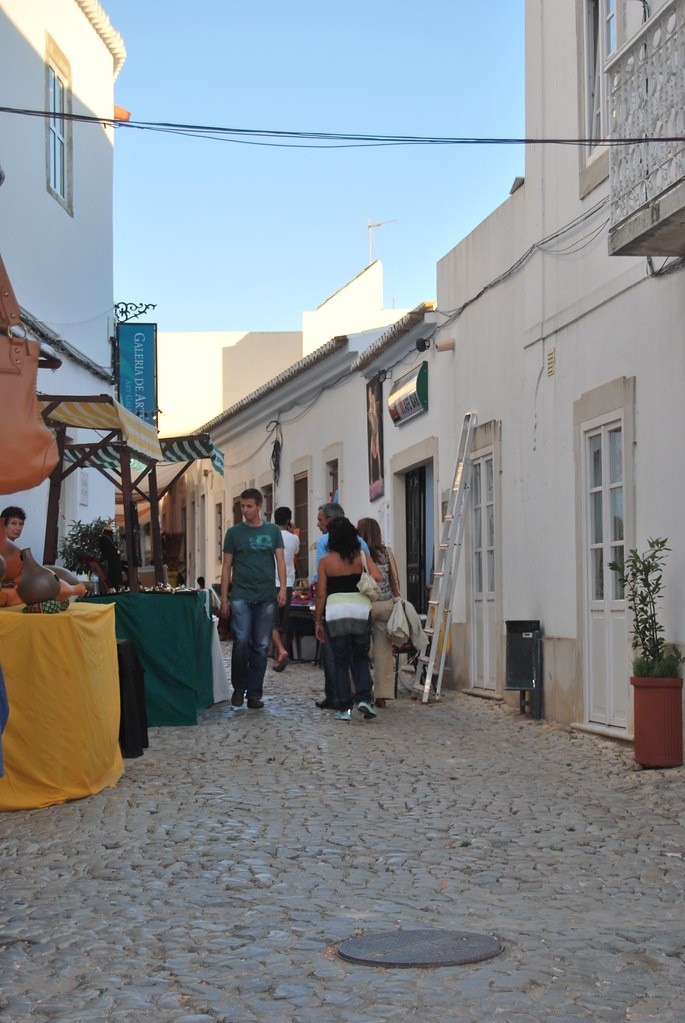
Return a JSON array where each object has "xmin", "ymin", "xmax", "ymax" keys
[{"xmin": 505, "ymin": 619, "xmax": 544, "ymax": 718}]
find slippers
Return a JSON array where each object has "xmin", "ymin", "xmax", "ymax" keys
[{"xmin": 272, "ymin": 657, "xmax": 290, "ymax": 672}]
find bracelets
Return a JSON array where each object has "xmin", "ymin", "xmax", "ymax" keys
[{"xmin": 314, "ymin": 621, "xmax": 323, "ymax": 626}]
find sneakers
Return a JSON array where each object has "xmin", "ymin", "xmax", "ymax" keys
[
  {"xmin": 358, "ymin": 701, "xmax": 376, "ymax": 719},
  {"xmin": 334, "ymin": 709, "xmax": 352, "ymax": 721}
]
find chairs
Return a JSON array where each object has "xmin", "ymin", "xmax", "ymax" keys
[{"xmin": 391, "ymin": 608, "xmax": 438, "ymax": 699}]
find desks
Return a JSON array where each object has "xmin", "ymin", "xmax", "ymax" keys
[
  {"xmin": 287, "ymin": 605, "xmax": 326, "ymax": 667},
  {"xmin": 0, "ymin": 588, "xmax": 233, "ymax": 811}
]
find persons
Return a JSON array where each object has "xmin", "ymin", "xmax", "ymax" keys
[
  {"xmin": 177, "ymin": 488, "xmax": 401, "ymax": 723},
  {"xmin": 0, "ymin": 507, "xmax": 26, "ymax": 542}
]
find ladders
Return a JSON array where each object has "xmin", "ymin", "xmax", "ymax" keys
[{"xmin": 412, "ymin": 412, "xmax": 478, "ymax": 706}]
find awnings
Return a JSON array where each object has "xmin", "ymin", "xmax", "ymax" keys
[{"xmin": 36, "ymin": 391, "xmax": 226, "ymax": 476}]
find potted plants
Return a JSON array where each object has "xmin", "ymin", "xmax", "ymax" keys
[{"xmin": 605, "ymin": 537, "xmax": 685, "ymax": 768}]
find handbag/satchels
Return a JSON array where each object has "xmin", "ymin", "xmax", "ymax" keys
[
  {"xmin": 356, "ymin": 549, "xmax": 381, "ymax": 602},
  {"xmin": 392, "ymin": 601, "xmax": 414, "ymax": 654}
]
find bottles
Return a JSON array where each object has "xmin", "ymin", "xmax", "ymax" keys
[
  {"xmin": 14, "ymin": 548, "xmax": 61, "ymax": 606},
  {"xmin": 42, "ymin": 565, "xmax": 95, "ymax": 604}
]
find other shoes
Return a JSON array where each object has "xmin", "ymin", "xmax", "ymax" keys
[
  {"xmin": 231, "ymin": 689, "xmax": 244, "ymax": 707},
  {"xmin": 316, "ymin": 699, "xmax": 331, "ymax": 708},
  {"xmin": 374, "ymin": 699, "xmax": 386, "ymax": 708},
  {"xmin": 247, "ymin": 699, "xmax": 264, "ymax": 708}
]
